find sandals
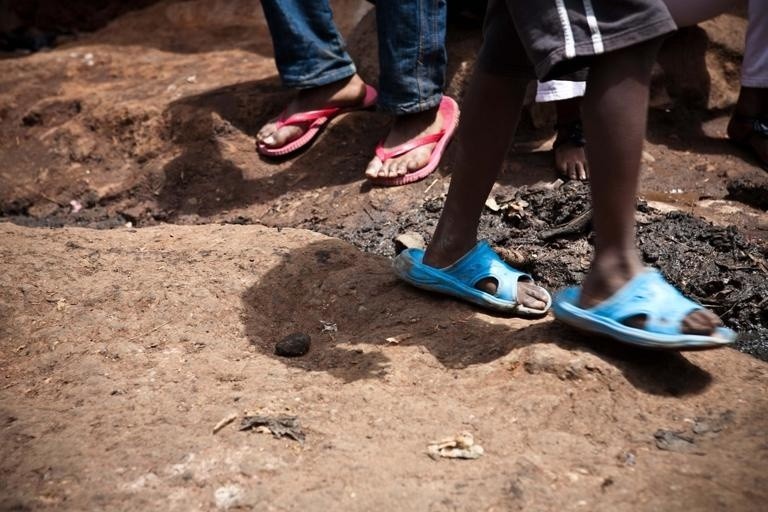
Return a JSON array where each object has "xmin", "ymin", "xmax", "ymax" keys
[
  {"xmin": 391, "ymin": 238, "xmax": 552, "ymax": 316},
  {"xmin": 553, "ymin": 268, "xmax": 738, "ymax": 351},
  {"xmin": 258, "ymin": 82, "xmax": 378, "ymax": 158},
  {"xmin": 370, "ymin": 96, "xmax": 460, "ymax": 186}
]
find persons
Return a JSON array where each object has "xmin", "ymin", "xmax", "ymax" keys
[
  {"xmin": 506, "ymin": 68, "xmax": 593, "ymax": 182},
  {"xmin": 663, "ymin": 1, "xmax": 768, "ymax": 167},
  {"xmin": 390, "ymin": 1, "xmax": 740, "ymax": 352},
  {"xmin": 254, "ymin": 0, "xmax": 460, "ymax": 187}
]
